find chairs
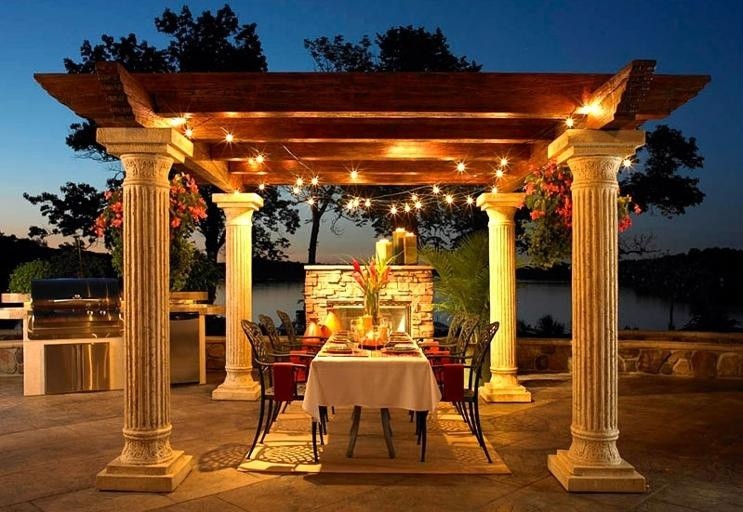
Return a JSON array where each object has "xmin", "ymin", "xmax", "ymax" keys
[
  {"xmin": 258, "ymin": 314, "xmax": 328, "ymax": 435},
  {"xmin": 411, "ymin": 316, "xmax": 480, "ymax": 435},
  {"xmin": 277, "ymin": 310, "xmax": 335, "ymax": 415},
  {"xmin": 409, "ymin": 311, "xmax": 466, "ymax": 415},
  {"xmin": 417, "ymin": 321, "xmax": 500, "ymax": 463},
  {"xmin": 241, "ymin": 319, "xmax": 324, "ymax": 463}
]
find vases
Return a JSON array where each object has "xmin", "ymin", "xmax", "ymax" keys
[{"xmin": 371, "ymin": 316, "xmax": 381, "ymax": 339}]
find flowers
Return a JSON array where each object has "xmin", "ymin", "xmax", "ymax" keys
[
  {"xmin": 339, "ymin": 250, "xmax": 404, "ymax": 328},
  {"xmin": 89, "ymin": 172, "xmax": 208, "ymax": 293},
  {"xmin": 516, "ymin": 162, "xmax": 642, "ymax": 271}
]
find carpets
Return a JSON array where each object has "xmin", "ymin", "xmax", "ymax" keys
[{"xmin": 238, "ymin": 400, "xmax": 511, "ymax": 475}]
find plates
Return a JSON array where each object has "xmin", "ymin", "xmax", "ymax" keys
[
  {"xmin": 335, "ymin": 333, "xmax": 347, "ymax": 334},
  {"xmin": 391, "ymin": 341, "xmax": 410, "ymax": 342},
  {"xmin": 331, "ymin": 340, "xmax": 347, "ymax": 342},
  {"xmin": 326, "ymin": 349, "xmax": 351, "ymax": 352},
  {"xmin": 392, "ymin": 335, "xmax": 405, "ymax": 335},
  {"xmin": 381, "ymin": 348, "xmax": 419, "ymax": 352}
]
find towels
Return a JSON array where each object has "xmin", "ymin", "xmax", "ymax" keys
[
  {"xmin": 304, "ymin": 338, "xmax": 319, "ymax": 354},
  {"xmin": 290, "ymin": 350, "xmax": 307, "ymax": 382},
  {"xmin": 443, "ymin": 364, "xmax": 464, "ymax": 402},
  {"xmin": 273, "ymin": 362, "xmax": 294, "ymax": 400},
  {"xmin": 424, "ymin": 341, "xmax": 440, "ymax": 353},
  {"xmin": 433, "ymin": 350, "xmax": 450, "ymax": 381}
]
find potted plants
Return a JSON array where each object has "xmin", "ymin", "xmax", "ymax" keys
[{"xmin": 412, "ymin": 230, "xmax": 490, "ymax": 389}]
